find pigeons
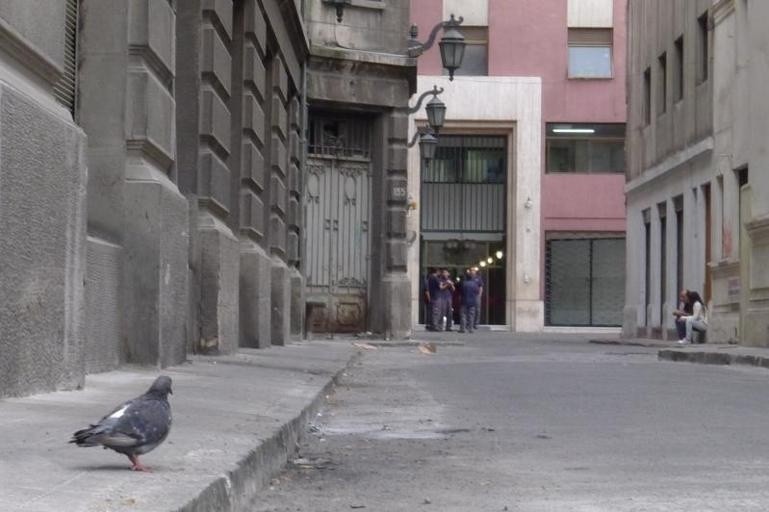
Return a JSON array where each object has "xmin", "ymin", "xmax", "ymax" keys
[{"xmin": 63, "ymin": 374, "xmax": 176, "ymax": 475}]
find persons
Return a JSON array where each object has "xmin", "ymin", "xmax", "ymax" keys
[
  {"xmin": 678, "ymin": 291, "xmax": 708, "ymax": 344},
  {"xmin": 676, "ymin": 289, "xmax": 694, "ymax": 343},
  {"xmin": 423, "ymin": 267, "xmax": 483, "ymax": 332}
]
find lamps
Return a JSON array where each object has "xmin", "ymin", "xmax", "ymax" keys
[
  {"xmin": 409, "ymin": 124, "xmax": 439, "ymax": 168},
  {"xmin": 409, "ymin": 85, "xmax": 446, "ymax": 135},
  {"xmin": 408, "ymin": 15, "xmax": 465, "ymax": 81}
]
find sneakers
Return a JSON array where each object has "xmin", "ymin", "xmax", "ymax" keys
[
  {"xmin": 678, "ymin": 338, "xmax": 693, "ymax": 345},
  {"xmin": 432, "ymin": 324, "xmax": 477, "ymax": 334}
]
